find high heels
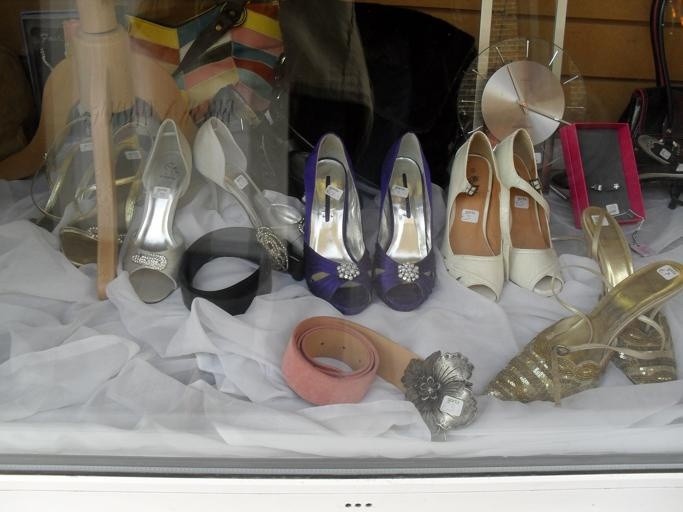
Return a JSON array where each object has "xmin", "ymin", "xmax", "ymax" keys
[
  {"xmin": 579, "ymin": 205, "xmax": 677, "ymax": 384},
  {"xmin": 29, "ymin": 117, "xmax": 564, "ymax": 315},
  {"xmin": 482, "ymin": 259, "xmax": 683, "ymax": 403}
]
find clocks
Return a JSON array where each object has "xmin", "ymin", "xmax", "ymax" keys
[{"xmin": 456, "ymin": 35, "xmax": 589, "ymax": 200}]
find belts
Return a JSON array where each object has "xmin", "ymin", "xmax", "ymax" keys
[{"xmin": 280, "ymin": 315, "xmax": 477, "ymax": 442}]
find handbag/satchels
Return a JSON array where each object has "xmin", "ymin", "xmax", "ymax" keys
[
  {"xmin": 620, "ymin": 0, "xmax": 683, "ymax": 183},
  {"xmin": 62, "ymin": 1, "xmax": 283, "ymax": 172}
]
